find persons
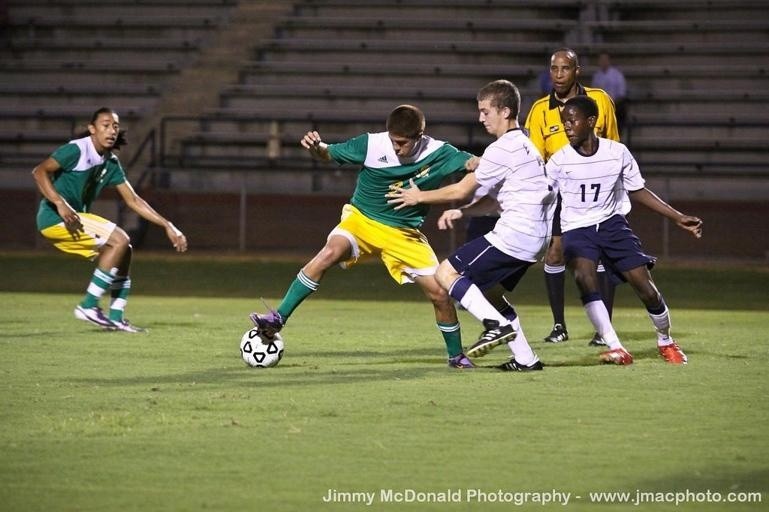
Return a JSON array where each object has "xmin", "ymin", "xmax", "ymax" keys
[
  {"xmin": 32, "ymin": 108, "xmax": 188, "ymax": 331},
  {"xmin": 591, "ymin": 52, "xmax": 628, "ymax": 131},
  {"xmin": 524, "ymin": 47, "xmax": 620, "ymax": 347},
  {"xmin": 250, "ymin": 104, "xmax": 479, "ymax": 369},
  {"xmin": 385, "ymin": 80, "xmax": 545, "ymax": 372},
  {"xmin": 545, "ymin": 96, "xmax": 703, "ymax": 363}
]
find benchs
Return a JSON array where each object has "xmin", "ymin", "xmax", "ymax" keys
[{"xmin": 0, "ymin": 0, "xmax": 239, "ymax": 166}]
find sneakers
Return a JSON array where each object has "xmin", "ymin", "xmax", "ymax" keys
[
  {"xmin": 544, "ymin": 324, "xmax": 568, "ymax": 343},
  {"xmin": 659, "ymin": 342, "xmax": 687, "ymax": 363},
  {"xmin": 590, "ymin": 333, "xmax": 632, "ymax": 364},
  {"xmin": 250, "ymin": 308, "xmax": 282, "ymax": 341},
  {"xmin": 75, "ymin": 304, "xmax": 142, "ymax": 332},
  {"xmin": 449, "ymin": 319, "xmax": 543, "ymax": 370}
]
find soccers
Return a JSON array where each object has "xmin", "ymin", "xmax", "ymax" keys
[{"xmin": 240, "ymin": 327, "xmax": 284, "ymax": 368}]
